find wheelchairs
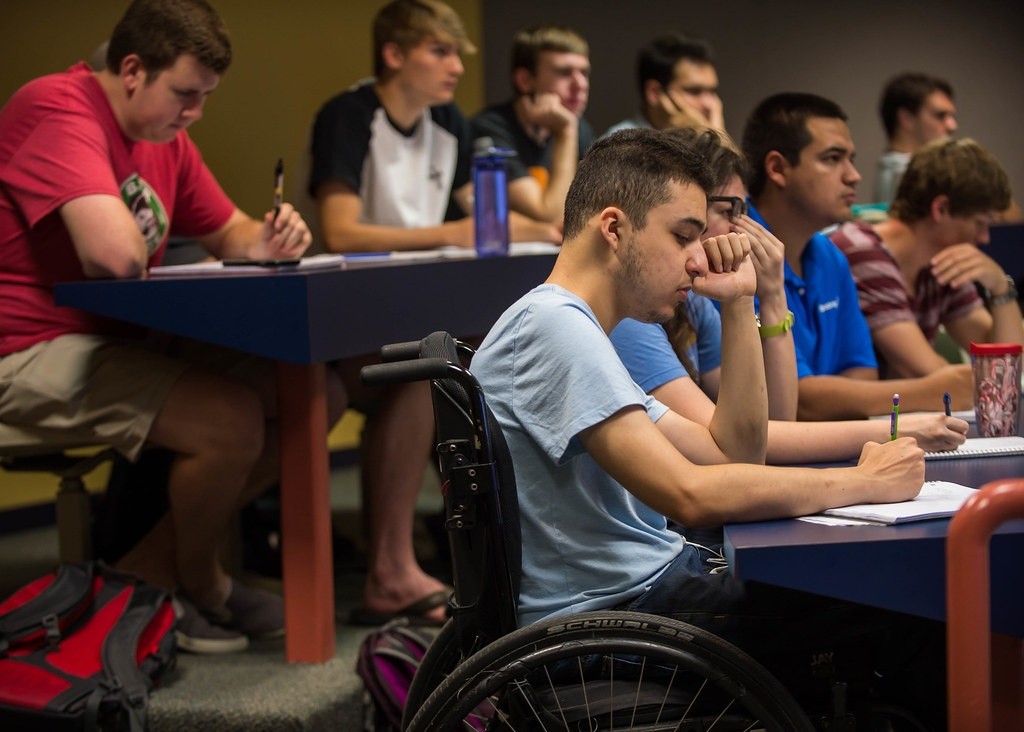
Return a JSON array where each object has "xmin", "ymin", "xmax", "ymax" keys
[{"xmin": 359, "ymin": 328, "xmax": 814, "ymax": 732}]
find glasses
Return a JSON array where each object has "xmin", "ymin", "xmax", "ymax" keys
[{"xmin": 707, "ymin": 196, "xmax": 748, "ymax": 219}]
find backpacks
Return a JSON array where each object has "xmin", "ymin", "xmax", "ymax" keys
[
  {"xmin": 356, "ymin": 625, "xmax": 495, "ymax": 732},
  {"xmin": 0, "ymin": 560, "xmax": 175, "ymax": 732}
]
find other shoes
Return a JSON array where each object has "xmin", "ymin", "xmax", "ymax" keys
[
  {"xmin": 174, "ymin": 597, "xmax": 250, "ymax": 653},
  {"xmin": 172, "ymin": 556, "xmax": 287, "ymax": 639}
]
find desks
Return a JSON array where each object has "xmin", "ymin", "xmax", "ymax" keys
[
  {"xmin": 48, "ymin": 235, "xmax": 559, "ymax": 662},
  {"xmin": 722, "ymin": 415, "xmax": 1024, "ymax": 732}
]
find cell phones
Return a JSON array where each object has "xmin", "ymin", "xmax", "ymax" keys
[{"xmin": 222, "ymin": 258, "xmax": 300, "ymax": 267}]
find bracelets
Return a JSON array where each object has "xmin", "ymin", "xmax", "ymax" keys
[
  {"xmin": 985, "ymin": 275, "xmax": 1017, "ymax": 306},
  {"xmin": 755, "ymin": 310, "xmax": 795, "ymax": 338}
]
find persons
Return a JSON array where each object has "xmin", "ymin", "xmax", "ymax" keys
[
  {"xmin": 874, "ymin": 73, "xmax": 1024, "ymax": 224},
  {"xmin": 307, "ymin": 0, "xmax": 562, "ymax": 624},
  {"xmin": 833, "ymin": 135, "xmax": 1024, "ymax": 377},
  {"xmin": 470, "ymin": 127, "xmax": 925, "ymax": 732},
  {"xmin": 472, "ymin": 18, "xmax": 591, "ymax": 221},
  {"xmin": 740, "ymin": 90, "xmax": 978, "ymax": 422},
  {"xmin": 1, "ymin": 0, "xmax": 312, "ymax": 653},
  {"xmin": 610, "ymin": 129, "xmax": 969, "ymax": 466},
  {"xmin": 599, "ymin": 40, "xmax": 726, "ymax": 141}
]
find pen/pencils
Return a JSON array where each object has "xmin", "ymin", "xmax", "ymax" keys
[
  {"xmin": 891, "ymin": 393, "xmax": 900, "ymax": 441},
  {"xmin": 943, "ymin": 392, "xmax": 951, "ymax": 417},
  {"xmin": 274, "ymin": 159, "xmax": 285, "ymax": 220}
]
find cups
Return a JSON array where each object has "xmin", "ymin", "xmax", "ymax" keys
[{"xmin": 969, "ymin": 341, "xmax": 1023, "ymax": 438}]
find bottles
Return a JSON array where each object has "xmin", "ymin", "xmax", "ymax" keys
[{"xmin": 472, "ymin": 148, "xmax": 518, "ymax": 256}]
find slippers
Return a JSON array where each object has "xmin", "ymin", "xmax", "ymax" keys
[{"xmin": 350, "ymin": 585, "xmax": 453, "ymax": 625}]
